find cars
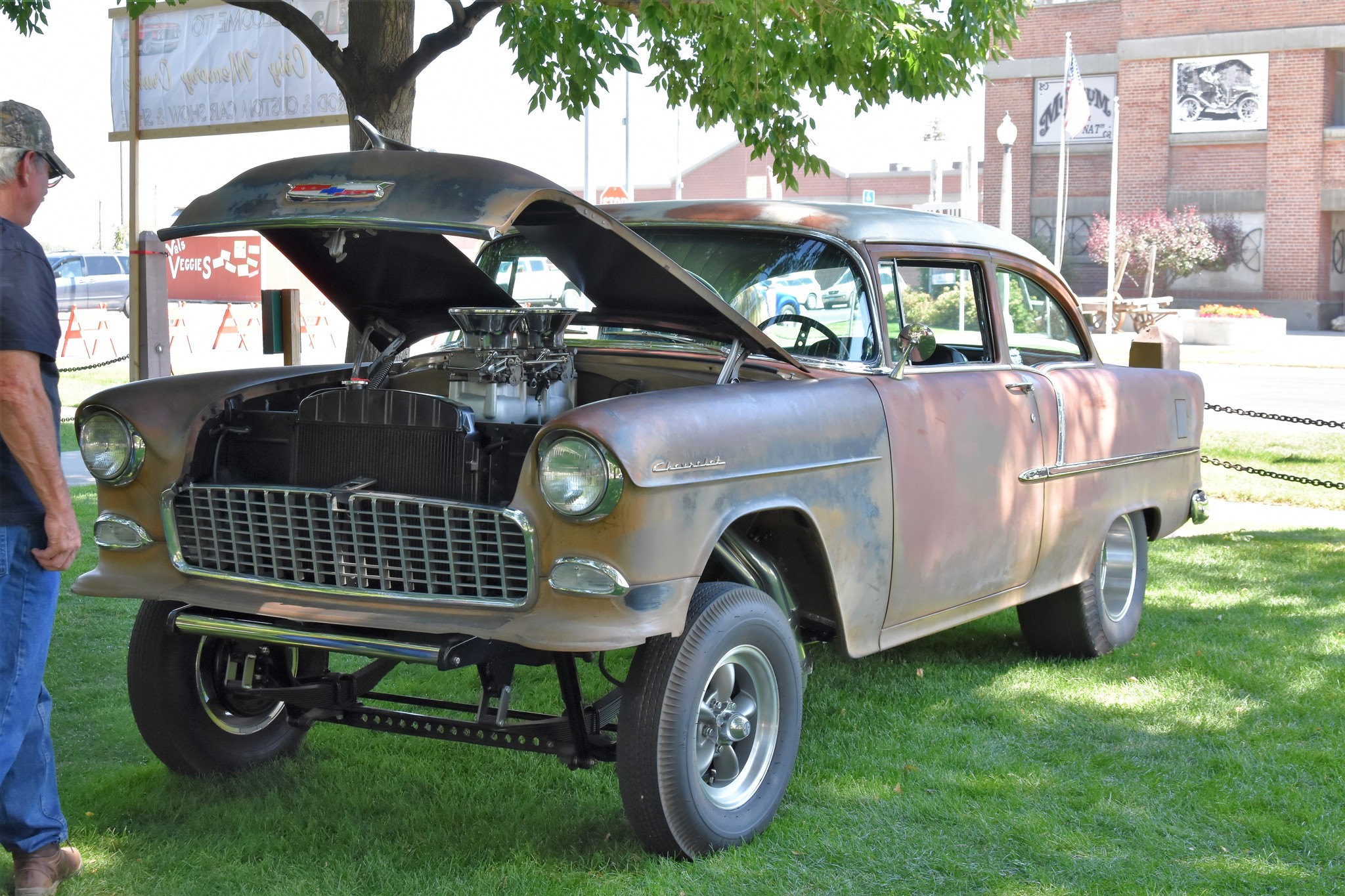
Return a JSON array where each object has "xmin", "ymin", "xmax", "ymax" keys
[
  {"xmin": 821, "ymin": 267, "xmax": 906, "ymax": 309},
  {"xmin": 67, "ymin": 116, "xmax": 1209, "ymax": 863},
  {"xmin": 734, "ymin": 271, "xmax": 823, "ymax": 328}
]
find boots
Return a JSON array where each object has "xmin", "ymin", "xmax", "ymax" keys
[{"xmin": 11, "ymin": 839, "xmax": 82, "ymax": 896}]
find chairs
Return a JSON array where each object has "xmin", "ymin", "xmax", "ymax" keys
[{"xmin": 808, "ymin": 337, "xmax": 969, "ymax": 365}]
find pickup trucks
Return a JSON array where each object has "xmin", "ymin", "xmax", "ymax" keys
[{"xmin": 490, "ymin": 251, "xmax": 585, "ymax": 309}]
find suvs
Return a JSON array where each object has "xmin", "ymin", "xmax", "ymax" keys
[{"xmin": 43, "ymin": 250, "xmax": 132, "ymax": 321}]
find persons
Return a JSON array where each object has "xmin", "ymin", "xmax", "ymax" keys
[{"xmin": 0, "ymin": 99, "xmax": 82, "ymax": 896}]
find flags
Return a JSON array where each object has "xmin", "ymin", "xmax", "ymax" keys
[{"xmin": 1063, "ymin": 51, "xmax": 1091, "ymax": 139}]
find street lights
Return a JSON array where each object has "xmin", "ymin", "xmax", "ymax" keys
[{"xmin": 996, "ymin": 110, "xmax": 1018, "ymax": 336}]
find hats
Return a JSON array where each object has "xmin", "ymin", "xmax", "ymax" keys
[{"xmin": 0, "ymin": 100, "xmax": 75, "ymax": 179}]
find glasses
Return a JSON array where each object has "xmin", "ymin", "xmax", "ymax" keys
[{"xmin": 20, "ymin": 150, "xmax": 64, "ymax": 188}]
